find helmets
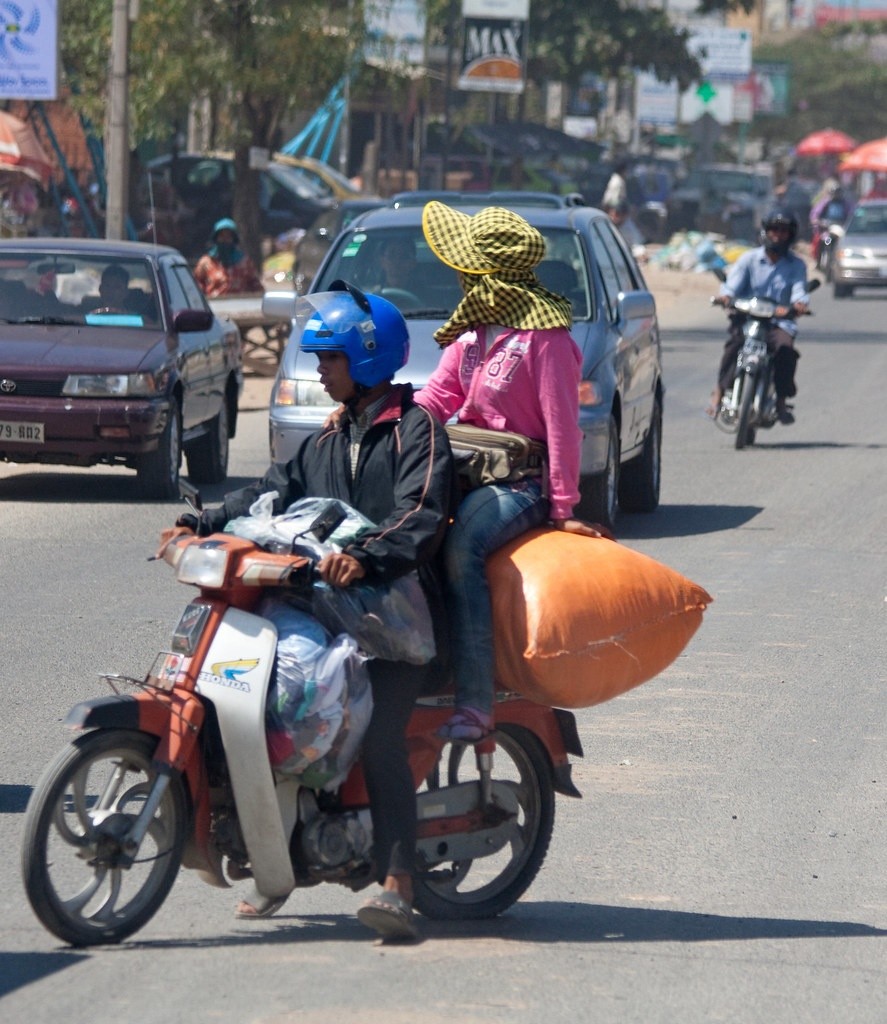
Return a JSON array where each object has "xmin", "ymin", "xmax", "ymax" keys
[
  {"xmin": 760, "ymin": 211, "xmax": 801, "ymax": 255},
  {"xmin": 300, "ymin": 288, "xmax": 409, "ymax": 389}
]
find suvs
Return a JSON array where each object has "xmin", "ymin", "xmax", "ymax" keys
[
  {"xmin": 668, "ymin": 162, "xmax": 775, "ymax": 240},
  {"xmin": 271, "ymin": 190, "xmax": 665, "ymax": 525}
]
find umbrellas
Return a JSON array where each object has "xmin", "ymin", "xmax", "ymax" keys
[
  {"xmin": 795, "ymin": 126, "xmax": 858, "ymax": 156},
  {"xmin": 836, "ymin": 134, "xmax": 887, "ymax": 186}
]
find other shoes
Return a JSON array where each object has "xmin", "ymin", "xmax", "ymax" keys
[
  {"xmin": 705, "ymin": 399, "xmax": 721, "ymax": 420},
  {"xmin": 777, "ymin": 407, "xmax": 796, "ymax": 424}
]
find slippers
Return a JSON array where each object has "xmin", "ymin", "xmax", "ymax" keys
[
  {"xmin": 235, "ymin": 886, "xmax": 284, "ymax": 919},
  {"xmin": 434, "ymin": 706, "xmax": 500, "ymax": 745},
  {"xmin": 358, "ymin": 894, "xmax": 421, "ymax": 942}
]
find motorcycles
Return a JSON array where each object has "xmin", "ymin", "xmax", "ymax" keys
[
  {"xmin": 809, "ymin": 221, "xmax": 846, "ymax": 271},
  {"xmin": 709, "ymin": 266, "xmax": 822, "ymax": 449},
  {"xmin": 20, "ymin": 480, "xmax": 582, "ymax": 946}
]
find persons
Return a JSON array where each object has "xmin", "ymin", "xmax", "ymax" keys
[
  {"xmin": 78, "ymin": 265, "xmax": 156, "ymax": 321},
  {"xmin": 365, "ymin": 237, "xmax": 425, "ymax": 306},
  {"xmin": 602, "ymin": 161, "xmax": 630, "ymax": 204},
  {"xmin": 808, "ymin": 177, "xmax": 854, "ymax": 269},
  {"xmin": 156, "ymin": 278, "xmax": 450, "ymax": 946},
  {"xmin": 769, "ymin": 160, "xmax": 809, "ymax": 239},
  {"xmin": 705, "ymin": 211, "xmax": 811, "ymax": 426},
  {"xmin": 192, "ymin": 218, "xmax": 265, "ymax": 299},
  {"xmin": 410, "ymin": 199, "xmax": 612, "ymax": 749}
]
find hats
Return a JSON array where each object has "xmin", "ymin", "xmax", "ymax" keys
[
  {"xmin": 208, "ymin": 218, "xmax": 243, "ymax": 265},
  {"xmin": 422, "ymin": 198, "xmax": 576, "ymax": 349}
]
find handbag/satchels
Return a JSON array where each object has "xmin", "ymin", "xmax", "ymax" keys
[
  {"xmin": 439, "ymin": 421, "xmax": 551, "ymax": 492},
  {"xmin": 221, "ymin": 492, "xmax": 437, "ymax": 797}
]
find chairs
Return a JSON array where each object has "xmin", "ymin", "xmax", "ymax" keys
[{"xmin": 537, "ymin": 260, "xmax": 577, "ymax": 295}]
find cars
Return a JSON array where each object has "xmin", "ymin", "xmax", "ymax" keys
[
  {"xmin": 830, "ymin": 197, "xmax": 887, "ymax": 297},
  {"xmin": 762, "ymin": 185, "xmax": 822, "ymax": 242},
  {"xmin": 1, "ymin": 236, "xmax": 244, "ymax": 501},
  {"xmin": 59, "ymin": 150, "xmax": 390, "ymax": 296}
]
